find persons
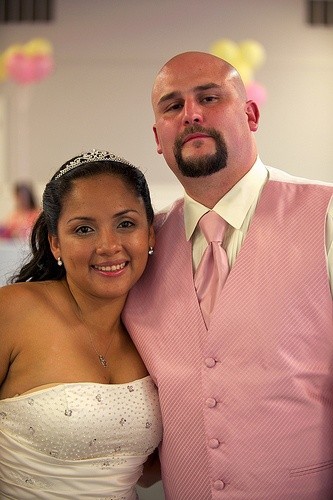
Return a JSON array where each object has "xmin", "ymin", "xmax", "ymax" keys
[
  {"xmin": 0, "ymin": 150, "xmax": 164, "ymax": 500},
  {"xmin": 7, "ymin": 182, "xmax": 42, "ymax": 241},
  {"xmin": 119, "ymin": 51, "xmax": 333, "ymax": 500}
]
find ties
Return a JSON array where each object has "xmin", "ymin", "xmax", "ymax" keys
[{"xmin": 193, "ymin": 211, "xmax": 229, "ymax": 331}]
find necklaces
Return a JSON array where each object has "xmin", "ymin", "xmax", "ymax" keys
[{"xmin": 79, "ymin": 308, "xmax": 115, "ymax": 368}]
